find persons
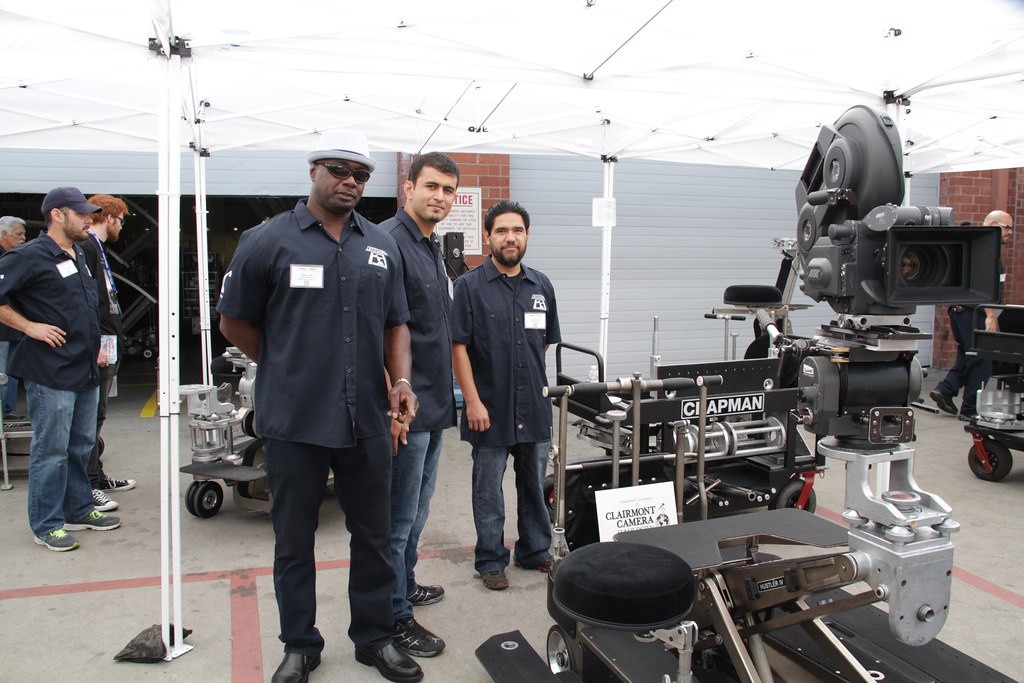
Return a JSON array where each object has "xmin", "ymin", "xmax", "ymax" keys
[
  {"xmin": 0, "ymin": 186, "xmax": 121, "ymax": 552},
  {"xmin": 76, "ymin": 194, "xmax": 136, "ymax": 512},
  {"xmin": 449, "ymin": 200, "xmax": 561, "ymax": 590},
  {"xmin": 216, "ymin": 128, "xmax": 425, "ymax": 683},
  {"xmin": 375, "ymin": 151, "xmax": 459, "ymax": 658},
  {"xmin": 0, "ymin": 216, "xmax": 28, "ymax": 421},
  {"xmin": 930, "ymin": 210, "xmax": 1013, "ymax": 422}
]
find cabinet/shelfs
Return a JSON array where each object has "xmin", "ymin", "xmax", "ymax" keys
[{"xmin": 181, "ymin": 251, "xmax": 221, "ymax": 321}]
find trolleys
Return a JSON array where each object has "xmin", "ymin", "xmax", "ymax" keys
[{"xmin": 963, "ymin": 305, "xmax": 1024, "ymax": 482}]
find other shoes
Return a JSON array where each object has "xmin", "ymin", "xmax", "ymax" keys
[
  {"xmin": 959, "ymin": 411, "xmax": 976, "ymax": 421},
  {"xmin": 3, "ymin": 413, "xmax": 26, "ymax": 420},
  {"xmin": 929, "ymin": 388, "xmax": 958, "ymax": 414}
]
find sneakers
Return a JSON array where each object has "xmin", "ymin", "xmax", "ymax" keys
[
  {"xmin": 63, "ymin": 508, "xmax": 121, "ymax": 532},
  {"xmin": 513, "ymin": 553, "xmax": 554, "ymax": 572},
  {"xmin": 99, "ymin": 474, "xmax": 136, "ymax": 491},
  {"xmin": 35, "ymin": 527, "xmax": 79, "ymax": 551},
  {"xmin": 406, "ymin": 582, "xmax": 445, "ymax": 605},
  {"xmin": 91, "ymin": 488, "xmax": 119, "ymax": 510},
  {"xmin": 481, "ymin": 568, "xmax": 510, "ymax": 590},
  {"xmin": 390, "ymin": 615, "xmax": 446, "ymax": 657}
]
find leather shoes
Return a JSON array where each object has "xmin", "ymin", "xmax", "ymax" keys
[
  {"xmin": 353, "ymin": 638, "xmax": 424, "ymax": 683},
  {"xmin": 271, "ymin": 651, "xmax": 321, "ymax": 683}
]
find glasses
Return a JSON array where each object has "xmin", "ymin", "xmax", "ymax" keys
[
  {"xmin": 992, "ymin": 221, "xmax": 1013, "ymax": 232},
  {"xmin": 313, "ymin": 161, "xmax": 370, "ymax": 184},
  {"xmin": 114, "ymin": 216, "xmax": 125, "ymax": 225}
]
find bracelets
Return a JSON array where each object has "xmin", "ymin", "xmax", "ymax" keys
[{"xmin": 393, "ymin": 378, "xmax": 412, "ymax": 390}]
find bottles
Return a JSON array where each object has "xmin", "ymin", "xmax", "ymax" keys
[
  {"xmin": 108, "ymin": 374, "xmax": 117, "ymax": 397},
  {"xmin": 588, "ymin": 366, "xmax": 599, "ymax": 383}
]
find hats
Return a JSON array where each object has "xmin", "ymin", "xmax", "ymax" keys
[
  {"xmin": 41, "ymin": 186, "xmax": 103, "ymax": 214},
  {"xmin": 306, "ymin": 129, "xmax": 377, "ymax": 173}
]
